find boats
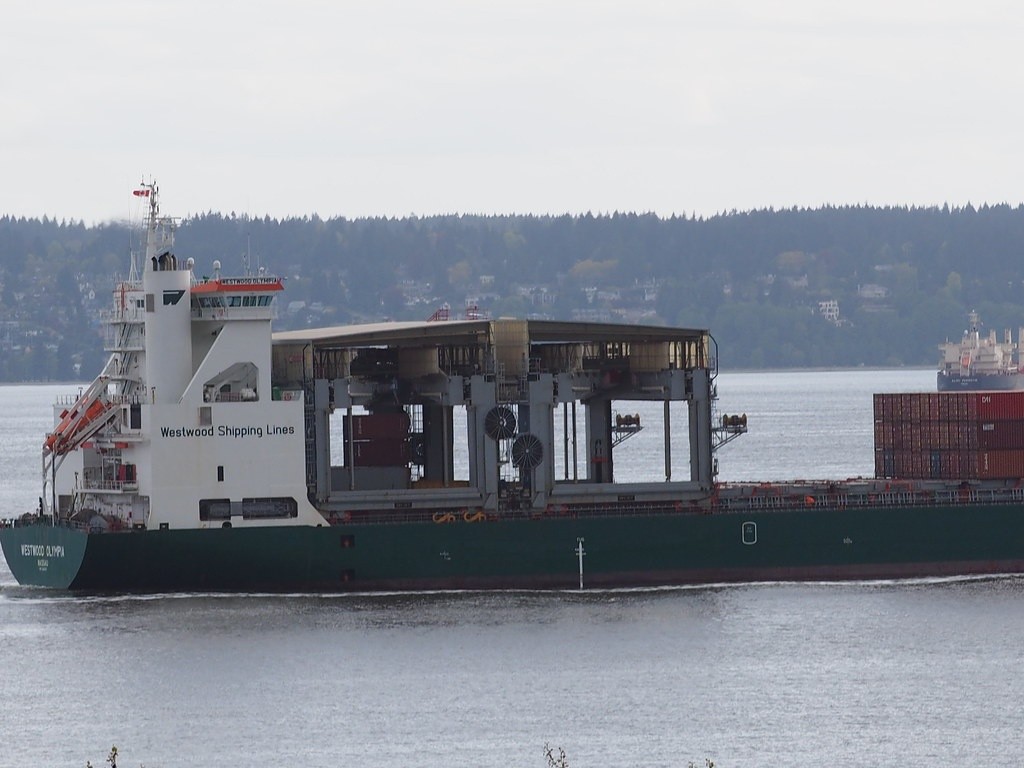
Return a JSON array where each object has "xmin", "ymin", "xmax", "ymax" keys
[
  {"xmin": 937, "ymin": 309, "xmax": 1024, "ymax": 391},
  {"xmin": 47, "ymin": 399, "xmax": 112, "ymax": 455},
  {"xmin": 0, "ymin": 174, "xmax": 1024, "ymax": 596}
]
